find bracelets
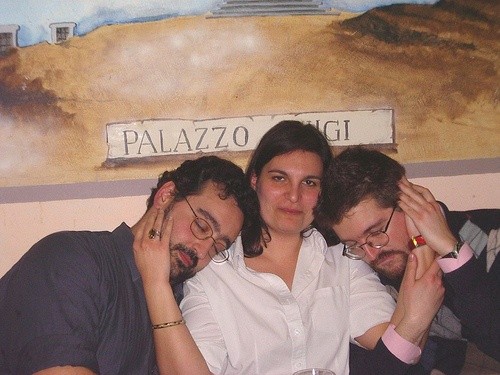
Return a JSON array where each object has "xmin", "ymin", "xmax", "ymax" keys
[
  {"xmin": 150, "ymin": 320, "xmax": 188, "ymax": 329},
  {"xmin": 407, "ymin": 233, "xmax": 431, "ymax": 251}
]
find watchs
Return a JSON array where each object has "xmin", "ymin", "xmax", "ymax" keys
[{"xmin": 441, "ymin": 236, "xmax": 465, "ymax": 258}]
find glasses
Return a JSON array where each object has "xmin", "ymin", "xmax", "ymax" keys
[
  {"xmin": 182, "ymin": 195, "xmax": 230, "ymax": 263},
  {"xmin": 342, "ymin": 207, "xmax": 395, "ymax": 260}
]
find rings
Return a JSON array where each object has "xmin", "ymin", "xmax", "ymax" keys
[{"xmin": 146, "ymin": 228, "xmax": 160, "ymax": 239}]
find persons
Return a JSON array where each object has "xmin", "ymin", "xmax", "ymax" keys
[
  {"xmin": 133, "ymin": 120, "xmax": 442, "ymax": 375},
  {"xmin": 33, "ymin": 155, "xmax": 272, "ymax": 375},
  {"xmin": 311, "ymin": 145, "xmax": 500, "ymax": 374}
]
